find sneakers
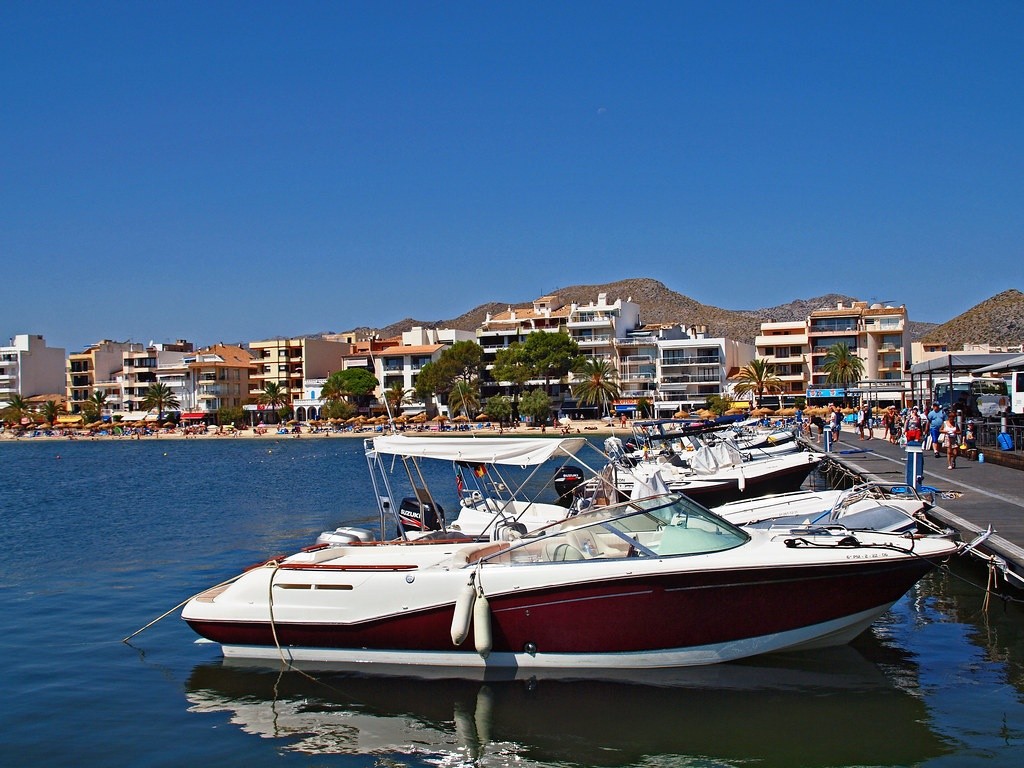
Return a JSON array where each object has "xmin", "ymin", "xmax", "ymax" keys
[
  {"xmin": 934, "ymin": 450, "xmax": 942, "ymax": 458},
  {"xmin": 952, "ymin": 461, "xmax": 956, "ymax": 468},
  {"xmin": 948, "ymin": 465, "xmax": 952, "ymax": 470}
]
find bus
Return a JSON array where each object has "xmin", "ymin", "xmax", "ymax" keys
[{"xmin": 932, "ymin": 377, "xmax": 1009, "ymax": 417}]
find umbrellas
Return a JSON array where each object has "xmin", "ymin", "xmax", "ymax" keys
[{"xmin": 674, "ymin": 406, "xmax": 795, "ymax": 418}]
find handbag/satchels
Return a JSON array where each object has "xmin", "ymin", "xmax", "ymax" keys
[
  {"xmin": 959, "ymin": 443, "xmax": 967, "ymax": 450},
  {"xmin": 921, "ymin": 432, "xmax": 933, "ymax": 452},
  {"xmin": 937, "ymin": 432, "xmax": 946, "ymax": 444},
  {"xmin": 900, "ymin": 434, "xmax": 907, "ymax": 448}
]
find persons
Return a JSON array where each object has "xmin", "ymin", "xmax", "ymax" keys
[
  {"xmin": 938, "ymin": 413, "xmax": 962, "ymax": 470},
  {"xmin": 0, "ymin": 413, "xmax": 303, "ymax": 440},
  {"xmin": 795, "ymin": 402, "xmax": 930, "ymax": 443},
  {"xmin": 925, "ymin": 401, "xmax": 949, "ymax": 458},
  {"xmin": 304, "ymin": 411, "xmax": 630, "ymax": 437},
  {"xmin": 961, "ymin": 420, "xmax": 979, "ymax": 461}
]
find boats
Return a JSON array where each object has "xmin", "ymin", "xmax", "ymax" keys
[
  {"xmin": 182, "ymin": 349, "xmax": 964, "ymax": 668},
  {"xmin": 395, "ymin": 382, "xmax": 937, "ymax": 542},
  {"xmin": 554, "ymin": 405, "xmax": 827, "ymax": 510}
]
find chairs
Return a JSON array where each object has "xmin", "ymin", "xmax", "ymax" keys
[{"xmin": 566, "ymin": 529, "xmax": 620, "ymax": 559}]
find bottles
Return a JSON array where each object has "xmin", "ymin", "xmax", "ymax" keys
[
  {"xmin": 587, "ymin": 539, "xmax": 595, "ymax": 556},
  {"xmin": 584, "ymin": 543, "xmax": 591, "ymax": 555},
  {"xmin": 979, "ymin": 452, "xmax": 984, "ymax": 462}
]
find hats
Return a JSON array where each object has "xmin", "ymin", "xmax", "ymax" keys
[
  {"xmin": 967, "ymin": 420, "xmax": 975, "ymax": 425},
  {"xmin": 913, "ymin": 405, "xmax": 919, "ymax": 410},
  {"xmin": 933, "ymin": 401, "xmax": 940, "ymax": 406}
]
